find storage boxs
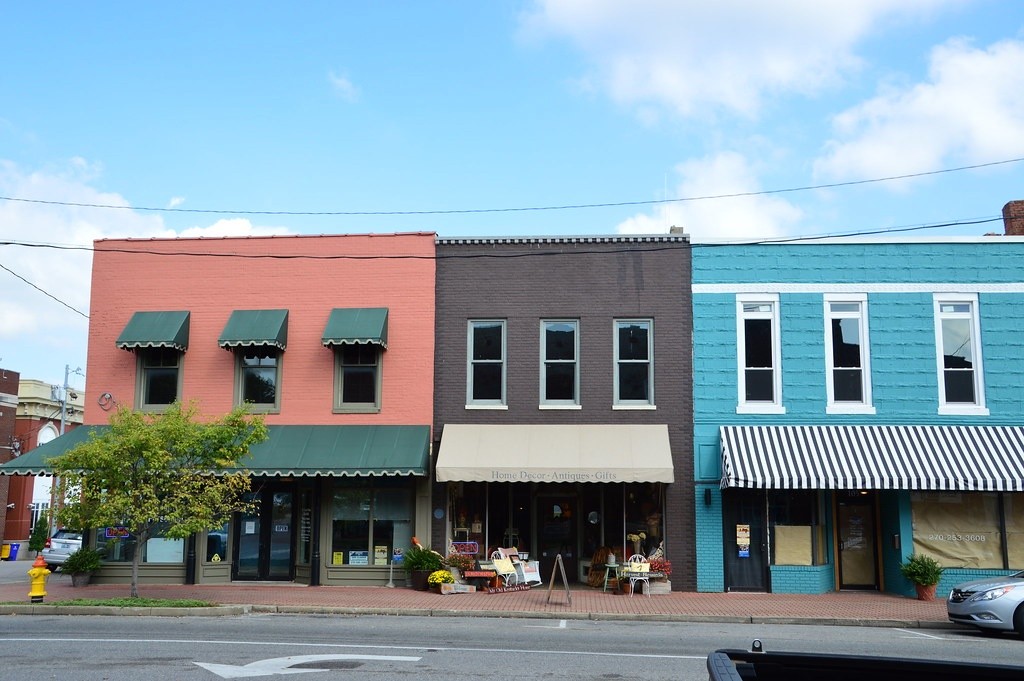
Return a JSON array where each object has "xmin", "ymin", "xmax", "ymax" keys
[{"xmin": 642, "ymin": 581, "xmax": 671, "ymax": 594}]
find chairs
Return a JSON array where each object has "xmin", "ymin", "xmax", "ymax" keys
[
  {"xmin": 628, "ymin": 553, "xmax": 651, "ymax": 598},
  {"xmin": 498, "ymin": 546, "xmax": 543, "ymax": 588},
  {"xmin": 491, "ymin": 551, "xmax": 518, "ymax": 588}
]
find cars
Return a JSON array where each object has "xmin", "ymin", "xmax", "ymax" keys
[
  {"xmin": 946, "ymin": 570, "xmax": 1024, "ymax": 638},
  {"xmin": 41, "ymin": 528, "xmax": 84, "ymax": 572}
]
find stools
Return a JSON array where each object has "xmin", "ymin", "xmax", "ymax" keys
[{"xmin": 603, "ymin": 563, "xmax": 619, "ymax": 592}]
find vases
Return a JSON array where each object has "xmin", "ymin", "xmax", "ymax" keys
[
  {"xmin": 653, "ymin": 577, "xmax": 667, "ymax": 583},
  {"xmin": 634, "ymin": 542, "xmax": 640, "ymax": 553},
  {"xmin": 393, "ymin": 555, "xmax": 403, "ymax": 565}
]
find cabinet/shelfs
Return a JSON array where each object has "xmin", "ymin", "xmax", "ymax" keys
[{"xmin": 453, "ymin": 527, "xmax": 471, "ymax": 542}]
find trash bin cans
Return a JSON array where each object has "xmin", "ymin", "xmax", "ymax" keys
[{"xmin": 9, "ymin": 543, "xmax": 20, "ymax": 561}]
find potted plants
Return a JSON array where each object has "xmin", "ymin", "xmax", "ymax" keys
[
  {"xmin": 59, "ymin": 545, "xmax": 107, "ymax": 586},
  {"xmin": 402, "ymin": 544, "xmax": 443, "ymax": 591},
  {"xmin": 623, "ymin": 576, "xmax": 632, "ymax": 593},
  {"xmin": 898, "ymin": 552, "xmax": 947, "ymax": 601},
  {"xmin": 609, "ymin": 573, "xmax": 617, "ymax": 587}
]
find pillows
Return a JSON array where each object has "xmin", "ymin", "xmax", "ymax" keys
[
  {"xmin": 631, "ymin": 562, "xmax": 650, "ymax": 577},
  {"xmin": 494, "ymin": 557, "xmax": 515, "ymax": 573},
  {"xmin": 508, "ymin": 553, "xmax": 520, "ymax": 563}
]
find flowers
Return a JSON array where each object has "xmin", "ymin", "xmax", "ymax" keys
[
  {"xmin": 394, "ymin": 547, "xmax": 404, "ymax": 555},
  {"xmin": 447, "ymin": 554, "xmax": 475, "ymax": 570},
  {"xmin": 627, "ymin": 532, "xmax": 646, "ymax": 543},
  {"xmin": 428, "ymin": 570, "xmax": 455, "ymax": 585},
  {"xmin": 648, "ymin": 557, "xmax": 672, "ymax": 579}
]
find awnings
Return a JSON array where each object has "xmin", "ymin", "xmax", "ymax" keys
[
  {"xmin": 435, "ymin": 423, "xmax": 676, "ymax": 484},
  {"xmin": 718, "ymin": 424, "xmax": 1024, "ymax": 492},
  {"xmin": 116, "ymin": 311, "xmax": 189, "ymax": 352},
  {"xmin": 0, "ymin": 425, "xmax": 430, "ymax": 480},
  {"xmin": 321, "ymin": 308, "xmax": 389, "ymax": 349},
  {"xmin": 218, "ymin": 309, "xmax": 290, "ymax": 351}
]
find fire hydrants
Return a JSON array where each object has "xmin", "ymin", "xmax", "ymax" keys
[{"xmin": 27, "ymin": 555, "xmax": 52, "ymax": 603}]
